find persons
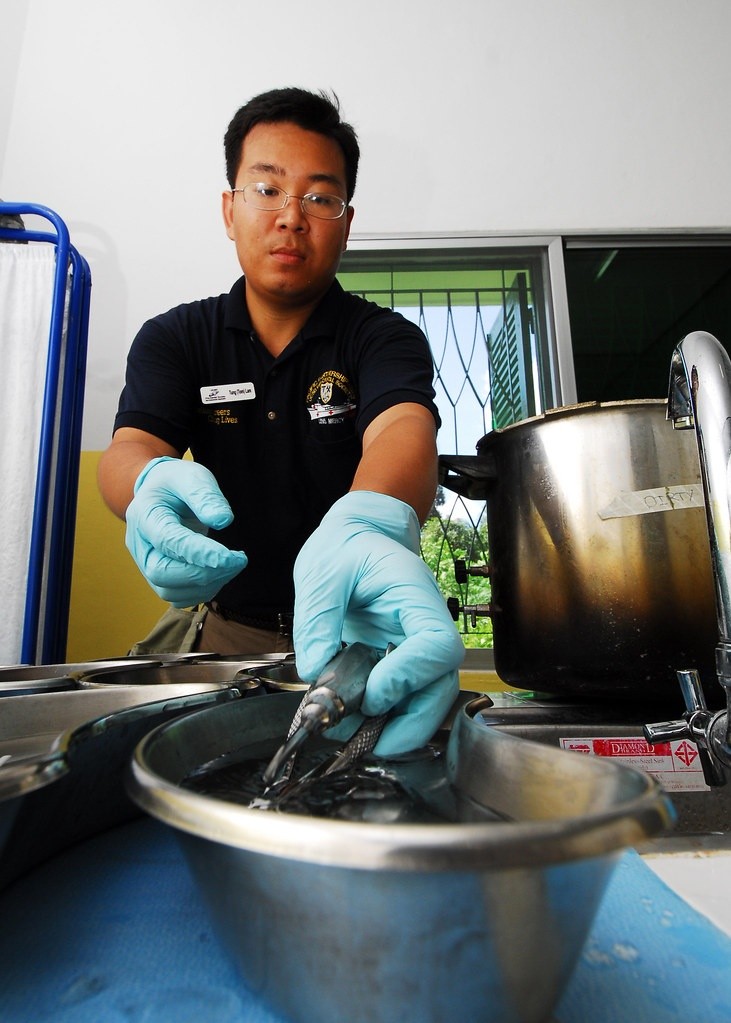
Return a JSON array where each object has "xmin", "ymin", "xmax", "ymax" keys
[{"xmin": 98, "ymin": 87, "xmax": 466, "ymax": 758}]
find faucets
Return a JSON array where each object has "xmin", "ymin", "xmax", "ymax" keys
[{"xmin": 644, "ymin": 331, "xmax": 731, "ymax": 788}]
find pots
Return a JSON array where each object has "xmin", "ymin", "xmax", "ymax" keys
[{"xmin": 435, "ymin": 396, "xmax": 722, "ymax": 707}]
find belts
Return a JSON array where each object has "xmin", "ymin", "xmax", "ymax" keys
[{"xmin": 206, "ymin": 601, "xmax": 294, "ymax": 636}]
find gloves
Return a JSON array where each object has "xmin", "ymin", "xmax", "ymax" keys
[
  {"xmin": 123, "ymin": 457, "xmax": 248, "ymax": 609},
  {"xmin": 290, "ymin": 490, "xmax": 467, "ymax": 756}
]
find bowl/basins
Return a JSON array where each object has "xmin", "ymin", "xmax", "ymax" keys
[{"xmin": 0, "ymin": 645, "xmax": 678, "ymax": 1023}]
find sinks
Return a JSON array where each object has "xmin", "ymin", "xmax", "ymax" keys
[{"xmin": 479, "ymin": 703, "xmax": 731, "ymax": 857}]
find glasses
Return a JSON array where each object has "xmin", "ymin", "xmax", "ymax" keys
[{"xmin": 230, "ymin": 182, "xmax": 348, "ymax": 220}]
